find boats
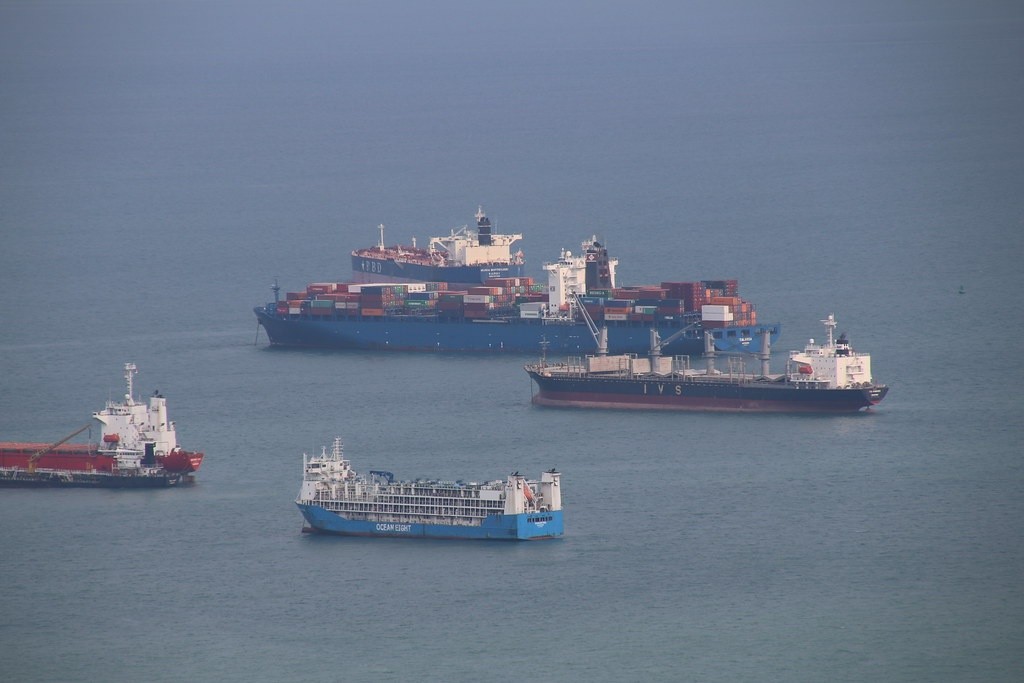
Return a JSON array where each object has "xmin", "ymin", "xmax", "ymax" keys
[
  {"xmin": 0, "ymin": 358, "xmax": 204, "ymax": 490},
  {"xmin": 162, "ymin": 450, "xmax": 204, "ymax": 473},
  {"xmin": 524, "ymin": 290, "xmax": 891, "ymax": 416},
  {"xmin": 294, "ymin": 425, "xmax": 572, "ymax": 541},
  {"xmin": 351, "ymin": 203, "xmax": 526, "ymax": 286},
  {"xmin": 104, "ymin": 434, "xmax": 119, "ymax": 442},
  {"xmin": 798, "ymin": 366, "xmax": 813, "ymax": 375}
]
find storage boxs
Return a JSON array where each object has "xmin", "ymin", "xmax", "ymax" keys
[{"xmin": 266, "ymin": 277, "xmax": 756, "ymax": 326}]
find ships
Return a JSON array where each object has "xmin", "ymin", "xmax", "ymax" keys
[{"xmin": 253, "ymin": 234, "xmax": 783, "ymax": 360}]
